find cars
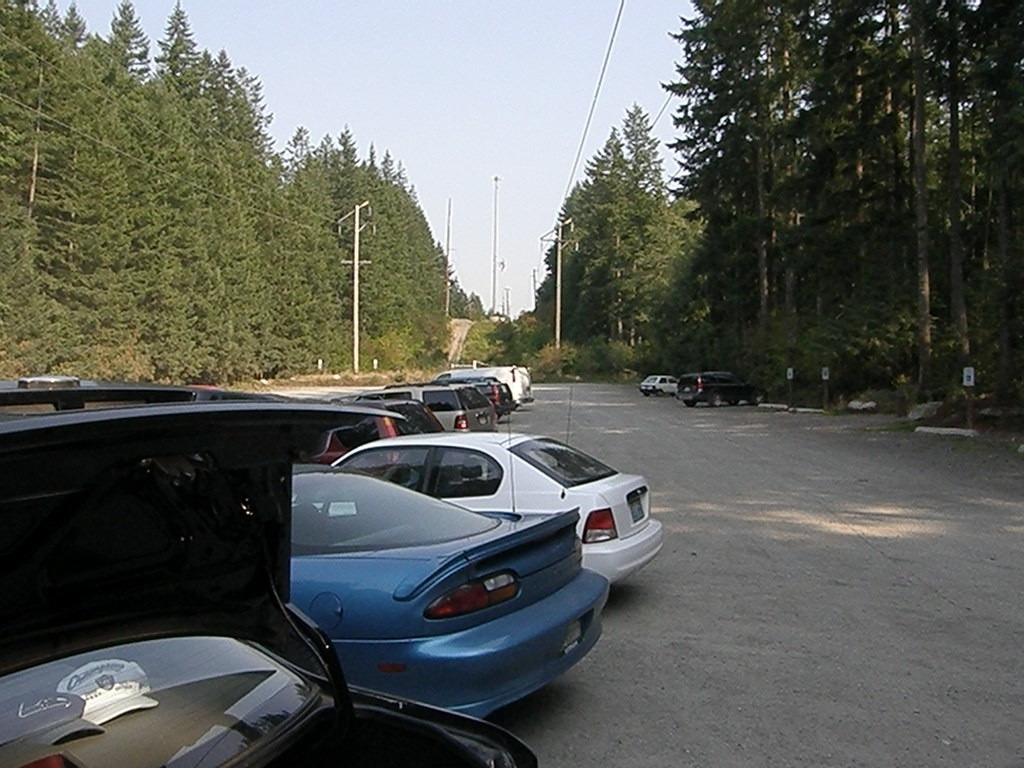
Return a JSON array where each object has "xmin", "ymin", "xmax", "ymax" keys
[
  {"xmin": 675, "ymin": 370, "xmax": 769, "ymax": 408},
  {"xmin": 0, "ymin": 366, "xmax": 665, "ymax": 767},
  {"xmin": 639, "ymin": 375, "xmax": 679, "ymax": 397}
]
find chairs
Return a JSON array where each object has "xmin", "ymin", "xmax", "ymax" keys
[{"xmin": 451, "ymin": 456, "xmax": 486, "ymax": 497}]
